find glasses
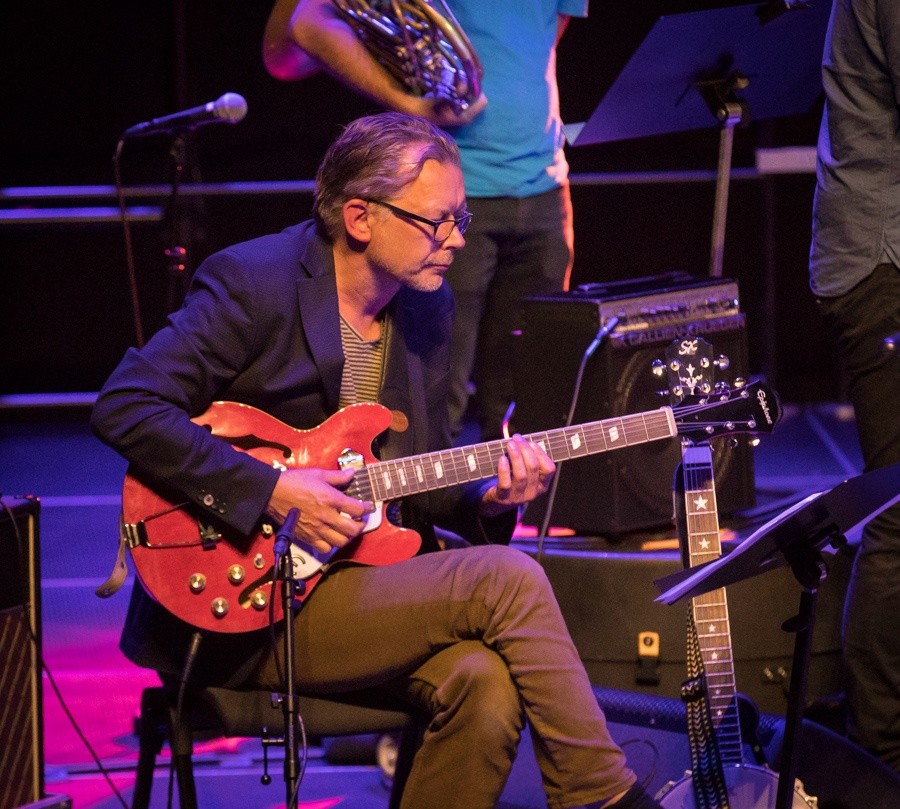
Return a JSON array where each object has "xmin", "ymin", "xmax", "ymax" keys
[{"xmin": 358, "ymin": 197, "xmax": 473, "ymax": 242}]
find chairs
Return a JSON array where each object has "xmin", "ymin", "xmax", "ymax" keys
[{"xmin": 128, "ymin": 518, "xmax": 477, "ymax": 809}]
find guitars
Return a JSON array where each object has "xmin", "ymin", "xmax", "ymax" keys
[
  {"xmin": 651, "ymin": 335, "xmax": 818, "ymax": 809},
  {"xmin": 121, "ymin": 376, "xmax": 783, "ymax": 635}
]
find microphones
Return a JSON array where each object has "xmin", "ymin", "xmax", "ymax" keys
[
  {"xmin": 125, "ymin": 92, "xmax": 248, "ymax": 141},
  {"xmin": 274, "ymin": 508, "xmax": 301, "ymax": 557}
]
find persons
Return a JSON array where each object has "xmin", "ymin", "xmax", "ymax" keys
[
  {"xmin": 808, "ymin": 0, "xmax": 900, "ymax": 774},
  {"xmin": 287, "ymin": 0, "xmax": 589, "ymax": 449},
  {"xmin": 92, "ymin": 114, "xmax": 665, "ymax": 809}
]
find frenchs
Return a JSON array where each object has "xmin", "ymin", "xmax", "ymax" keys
[{"xmin": 262, "ymin": 0, "xmax": 483, "ymax": 115}]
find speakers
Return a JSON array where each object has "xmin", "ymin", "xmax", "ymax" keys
[
  {"xmin": 0, "ymin": 493, "xmax": 43, "ymax": 809},
  {"xmin": 499, "ymin": 689, "xmax": 899, "ymax": 809},
  {"xmin": 513, "ymin": 267, "xmax": 752, "ymax": 534}
]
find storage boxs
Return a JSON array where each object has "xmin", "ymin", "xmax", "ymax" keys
[{"xmin": 511, "ymin": 531, "xmax": 839, "ymax": 726}]
